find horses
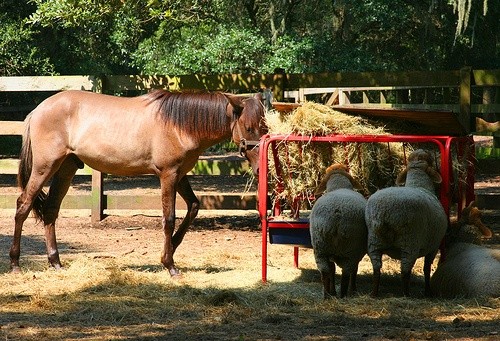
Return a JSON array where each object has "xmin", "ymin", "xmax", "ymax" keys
[{"xmin": 8, "ymin": 89, "xmax": 266, "ymax": 281}]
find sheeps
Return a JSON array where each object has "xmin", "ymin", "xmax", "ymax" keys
[
  {"xmin": 309, "ymin": 162, "xmax": 369, "ymax": 303},
  {"xmin": 431, "ymin": 206, "xmax": 500, "ymax": 297},
  {"xmin": 364, "ymin": 148, "xmax": 448, "ymax": 301}
]
